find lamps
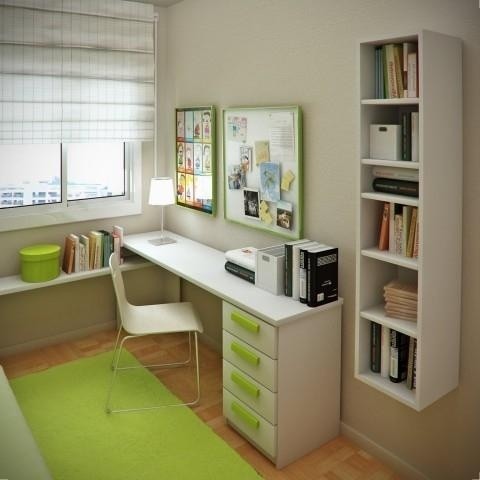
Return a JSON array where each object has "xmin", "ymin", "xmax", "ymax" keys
[{"xmin": 147, "ymin": 177, "xmax": 178, "ymax": 246}]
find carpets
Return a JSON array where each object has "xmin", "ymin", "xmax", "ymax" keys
[{"xmin": 7, "ymin": 347, "xmax": 266, "ymax": 480}]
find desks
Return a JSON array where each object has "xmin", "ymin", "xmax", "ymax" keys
[{"xmin": 120, "ymin": 229, "xmax": 347, "ymax": 471}]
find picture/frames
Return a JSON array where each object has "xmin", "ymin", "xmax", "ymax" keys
[{"xmin": 172, "ymin": 103, "xmax": 305, "ymax": 242}]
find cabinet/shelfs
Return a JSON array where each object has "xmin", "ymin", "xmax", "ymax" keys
[{"xmin": 353, "ymin": 26, "xmax": 466, "ymax": 414}]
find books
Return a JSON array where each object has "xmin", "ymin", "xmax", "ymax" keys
[
  {"xmin": 61, "ymin": 230, "xmax": 121, "ymax": 274},
  {"xmin": 284, "ymin": 238, "xmax": 338, "ymax": 307},
  {"xmin": 369, "ymin": 42, "xmax": 418, "ymax": 392}
]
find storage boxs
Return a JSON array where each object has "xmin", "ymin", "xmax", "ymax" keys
[{"xmin": 368, "ymin": 122, "xmax": 404, "ymax": 162}]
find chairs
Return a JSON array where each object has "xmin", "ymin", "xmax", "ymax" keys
[{"xmin": 105, "ymin": 250, "xmax": 205, "ymax": 414}]
